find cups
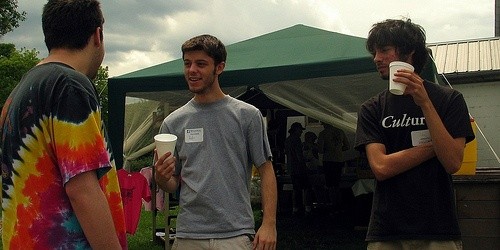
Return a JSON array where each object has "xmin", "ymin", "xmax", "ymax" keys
[
  {"xmin": 154, "ymin": 134, "xmax": 177, "ymax": 162},
  {"xmin": 389, "ymin": 61, "xmax": 414, "ymax": 95}
]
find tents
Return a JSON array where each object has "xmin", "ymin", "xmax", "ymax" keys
[{"xmin": 108, "ymin": 23, "xmax": 447, "ymax": 172}]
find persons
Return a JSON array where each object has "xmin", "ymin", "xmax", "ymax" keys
[
  {"xmin": 302, "ymin": 132, "xmax": 324, "ymax": 205},
  {"xmin": 151, "ymin": 35, "xmax": 277, "ymax": 250},
  {"xmin": 0, "ymin": 0, "xmax": 127, "ymax": 250},
  {"xmin": 304, "ymin": 121, "xmax": 349, "ymax": 208},
  {"xmin": 284, "ymin": 122, "xmax": 315, "ymax": 215},
  {"xmin": 355, "ymin": 18, "xmax": 474, "ymax": 249}
]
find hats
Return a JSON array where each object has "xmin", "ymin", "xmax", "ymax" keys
[{"xmin": 291, "ymin": 122, "xmax": 306, "ymax": 130}]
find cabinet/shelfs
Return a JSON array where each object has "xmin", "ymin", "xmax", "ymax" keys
[{"xmin": 151, "ymin": 102, "xmax": 180, "ymax": 250}]
